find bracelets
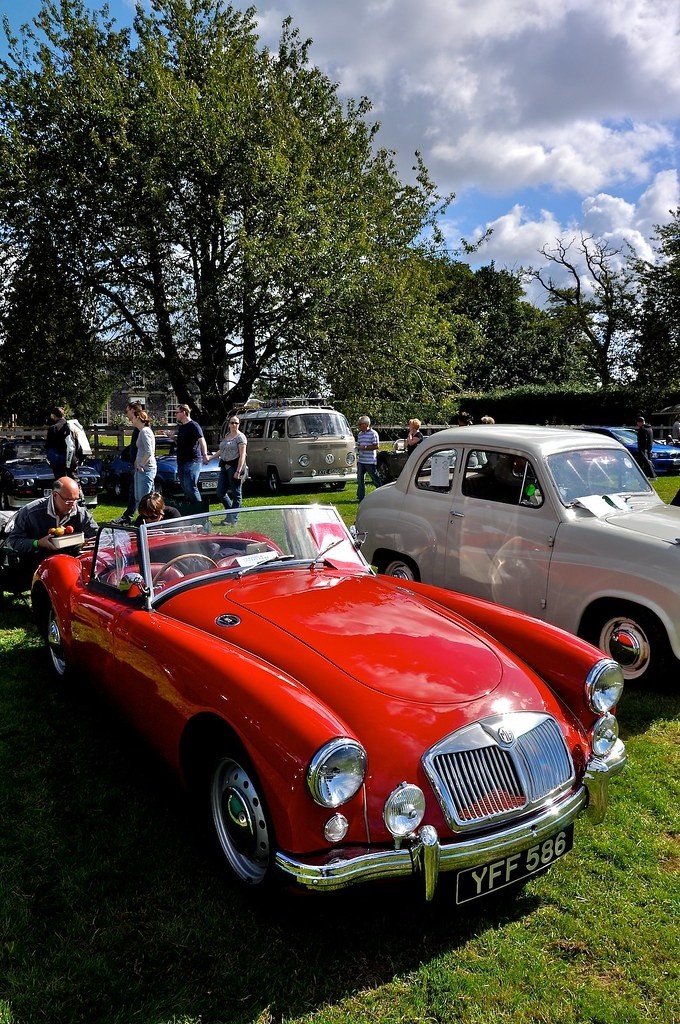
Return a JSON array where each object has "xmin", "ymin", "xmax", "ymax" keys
[
  {"xmin": 236, "ymin": 471, "xmax": 240, "ymax": 474},
  {"xmin": 364, "ymin": 445, "xmax": 367, "ymax": 450},
  {"xmin": 203, "ymin": 454, "xmax": 207, "ymax": 456},
  {"xmin": 32, "ymin": 540, "xmax": 39, "ymax": 548}
]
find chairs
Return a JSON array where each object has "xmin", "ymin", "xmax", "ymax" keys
[
  {"xmin": 462, "ymin": 473, "xmax": 527, "ymax": 505},
  {"xmin": 168, "ymin": 441, "xmax": 178, "ymax": 455},
  {"xmin": 210, "ymin": 547, "xmax": 246, "ymax": 568},
  {"xmin": 87, "ymin": 458, "xmax": 110, "ymax": 492},
  {"xmin": 105, "ymin": 562, "xmax": 185, "ymax": 587}
]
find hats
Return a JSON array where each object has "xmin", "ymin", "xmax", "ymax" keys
[{"xmin": 635, "ymin": 417, "xmax": 645, "ymax": 423}]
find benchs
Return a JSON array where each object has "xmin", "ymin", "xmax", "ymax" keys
[{"xmin": 416, "ymin": 480, "xmax": 453, "ymax": 492}]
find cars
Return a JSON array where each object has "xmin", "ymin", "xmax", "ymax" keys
[
  {"xmin": 1, "ymin": 440, "xmax": 102, "ymax": 511},
  {"xmin": 106, "ymin": 436, "xmax": 253, "ymax": 506},
  {"xmin": 573, "ymin": 424, "xmax": 680, "ymax": 477},
  {"xmin": 30, "ymin": 503, "xmax": 628, "ymax": 904},
  {"xmin": 512, "ymin": 450, "xmax": 617, "ymax": 478},
  {"xmin": 354, "ymin": 419, "xmax": 679, "ymax": 692},
  {"xmin": 377, "ymin": 436, "xmax": 487, "ymax": 484}
]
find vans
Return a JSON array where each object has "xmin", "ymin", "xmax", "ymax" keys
[{"xmin": 218, "ymin": 398, "xmax": 358, "ymax": 494}]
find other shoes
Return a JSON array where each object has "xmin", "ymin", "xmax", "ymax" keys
[
  {"xmin": 648, "ymin": 476, "xmax": 657, "ymax": 482},
  {"xmin": 351, "ymin": 499, "xmax": 359, "ymax": 502},
  {"xmin": 221, "ymin": 518, "xmax": 237, "ymax": 526}
]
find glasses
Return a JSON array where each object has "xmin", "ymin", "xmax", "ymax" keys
[
  {"xmin": 139, "ymin": 513, "xmax": 159, "ymax": 520},
  {"xmin": 126, "ymin": 408, "xmax": 132, "ymax": 414},
  {"xmin": 54, "ymin": 491, "xmax": 82, "ymax": 504},
  {"xmin": 175, "ymin": 410, "xmax": 184, "ymax": 414},
  {"xmin": 359, "ymin": 423, "xmax": 366, "ymax": 426},
  {"xmin": 229, "ymin": 421, "xmax": 240, "ymax": 425}
]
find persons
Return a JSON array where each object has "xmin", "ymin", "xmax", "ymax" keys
[
  {"xmin": 134, "ymin": 493, "xmax": 181, "ymax": 526},
  {"xmin": 205, "ymin": 415, "xmax": 247, "ymax": 528},
  {"xmin": 404, "ymin": 418, "xmax": 423, "ymax": 458},
  {"xmin": 45, "ymin": 400, "xmax": 170, "ymax": 526},
  {"xmin": 9, "ymin": 475, "xmax": 101, "ymax": 563},
  {"xmin": 637, "ymin": 417, "xmax": 658, "ymax": 479},
  {"xmin": 458, "ymin": 411, "xmax": 495, "ymax": 468},
  {"xmin": 174, "ymin": 405, "xmax": 209, "ymax": 502},
  {"xmin": 351, "ymin": 416, "xmax": 382, "ymax": 502},
  {"xmin": 666, "ymin": 415, "xmax": 680, "ymax": 448}
]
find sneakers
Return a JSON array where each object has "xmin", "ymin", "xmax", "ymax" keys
[{"xmin": 113, "ymin": 516, "xmax": 132, "ymax": 526}]
